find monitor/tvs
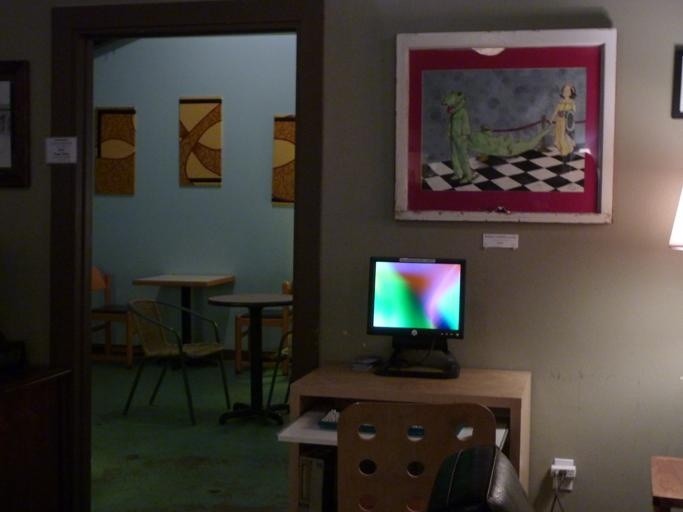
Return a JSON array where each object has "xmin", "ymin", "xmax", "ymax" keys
[{"xmin": 367, "ymin": 256, "xmax": 466, "ymax": 379}]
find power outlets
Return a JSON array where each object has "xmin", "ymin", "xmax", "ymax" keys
[{"xmin": 550, "ymin": 455, "xmax": 577, "ymax": 492}]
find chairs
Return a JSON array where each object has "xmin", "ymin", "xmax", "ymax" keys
[
  {"xmin": 264, "ymin": 330, "xmax": 293, "ymax": 411},
  {"xmin": 327, "ymin": 397, "xmax": 500, "ymax": 511},
  {"xmin": 116, "ymin": 297, "xmax": 232, "ymax": 428},
  {"xmin": 231, "ymin": 277, "xmax": 295, "ymax": 377},
  {"xmin": 90, "ymin": 264, "xmax": 142, "ymax": 369},
  {"xmin": 429, "ymin": 441, "xmax": 538, "ymax": 511}
]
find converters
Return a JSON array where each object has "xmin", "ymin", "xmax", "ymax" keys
[{"xmin": 551, "ymin": 465, "xmax": 576, "ymax": 478}]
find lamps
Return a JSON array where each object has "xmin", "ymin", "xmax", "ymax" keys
[{"xmin": 663, "ymin": 190, "xmax": 683, "ymax": 253}]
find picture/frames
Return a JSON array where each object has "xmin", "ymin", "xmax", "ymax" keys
[
  {"xmin": 668, "ymin": 45, "xmax": 683, "ymax": 118},
  {"xmin": 390, "ymin": 24, "xmax": 621, "ymax": 229},
  {"xmin": 0, "ymin": 55, "xmax": 38, "ymax": 193}
]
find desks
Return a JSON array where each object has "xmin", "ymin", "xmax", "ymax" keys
[
  {"xmin": 273, "ymin": 356, "xmax": 535, "ymax": 511},
  {"xmin": 129, "ymin": 268, "xmax": 236, "ymax": 373},
  {"xmin": 206, "ymin": 289, "xmax": 293, "ymax": 430},
  {"xmin": 647, "ymin": 450, "xmax": 683, "ymax": 511}
]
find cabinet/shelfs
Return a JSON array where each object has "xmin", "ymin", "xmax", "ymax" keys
[{"xmin": 0, "ymin": 358, "xmax": 77, "ymax": 512}]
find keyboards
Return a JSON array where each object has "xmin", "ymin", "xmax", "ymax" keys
[{"xmin": 319, "ymin": 407, "xmax": 466, "ymax": 437}]
